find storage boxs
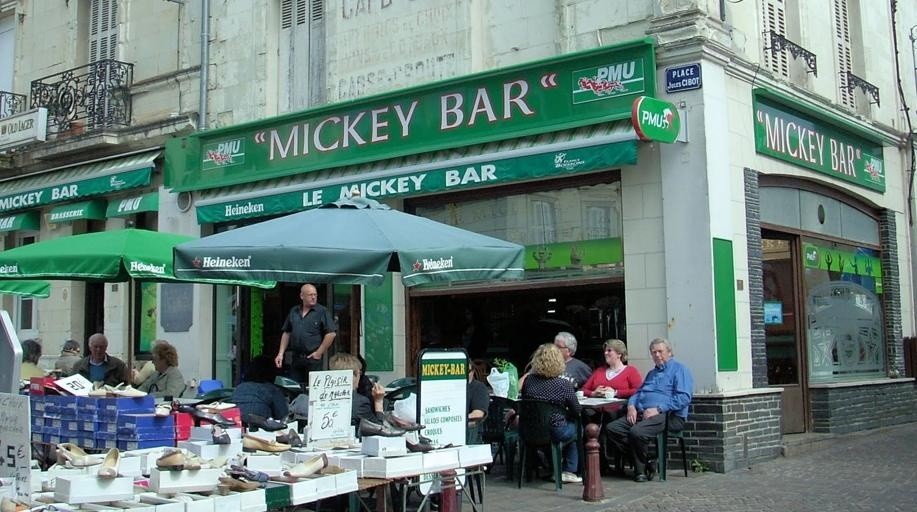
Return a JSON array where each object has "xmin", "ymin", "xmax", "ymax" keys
[
  {"xmin": 60, "ymin": 431, "xmax": 77, "ymax": 447},
  {"xmin": 78, "ymin": 396, "xmax": 98, "ymax": 414},
  {"xmin": 43, "ymin": 411, "xmax": 62, "ymax": 428},
  {"xmin": 62, "ymin": 414, "xmax": 78, "ymax": 430},
  {"xmin": 324, "ymin": 452, "xmax": 361, "ymax": 473},
  {"xmin": 44, "ymin": 394, "xmax": 63, "ymax": 414},
  {"xmin": 116, "ymin": 413, "xmax": 174, "ymax": 442},
  {"xmin": 172, "ymin": 407, "xmax": 242, "ymax": 440},
  {"xmin": 213, "ymin": 490, "xmax": 266, "ymax": 511},
  {"xmin": 267, "ymin": 477, "xmax": 317, "ymax": 504},
  {"xmin": 118, "ymin": 497, "xmax": 185, "ymax": 512},
  {"xmin": 302, "ymin": 426, "xmax": 354, "ymax": 449},
  {"xmin": 81, "ymin": 500, "xmax": 153, "ymax": 511},
  {"xmin": 362, "ymin": 435, "xmax": 406, "ymax": 456},
  {"xmin": 30, "ymin": 425, "xmax": 44, "ymax": 443},
  {"xmin": 98, "ymin": 395, "xmax": 155, "ymax": 417},
  {"xmin": 125, "ymin": 446, "xmax": 187, "ymax": 475},
  {"xmin": 116, "ymin": 456, "xmax": 141, "ymax": 479},
  {"xmin": 363, "ymin": 455, "xmax": 423, "ymax": 480},
  {"xmin": 283, "ymin": 446, "xmax": 348, "ymax": 468},
  {"xmin": 340, "ymin": 455, "xmax": 366, "ymax": 478},
  {"xmin": 118, "ymin": 440, "xmax": 175, "ymax": 453},
  {"xmin": 186, "ymin": 492, "xmax": 240, "ymax": 511},
  {"xmin": 245, "ymin": 422, "xmax": 299, "ymax": 445},
  {"xmin": 191, "ymin": 441, "xmax": 243, "ymax": 463},
  {"xmin": 190, "ymin": 425, "xmax": 242, "ymax": 443},
  {"xmin": 77, "ymin": 414, "xmax": 98, "ymax": 433},
  {"xmin": 77, "ymin": 430, "xmax": 95, "ymax": 449},
  {"xmin": 317, "ymin": 468, "xmax": 359, "ymax": 494},
  {"xmin": 243, "ymin": 452, "xmax": 283, "ymax": 475},
  {"xmin": 42, "ymin": 427, "xmax": 61, "ymax": 443},
  {"xmin": 31, "ymin": 411, "xmax": 45, "ymax": 426},
  {"xmin": 88, "ymin": 465, "xmax": 102, "ymax": 474},
  {"xmin": 48, "ymin": 458, "xmax": 86, "ymax": 483},
  {"xmin": 437, "ymin": 443, "xmax": 492, "ymax": 467},
  {"xmin": 141, "ymin": 491, "xmax": 215, "ymax": 512},
  {"xmin": 242, "ymin": 480, "xmax": 292, "ymax": 507},
  {"xmin": 97, "ymin": 432, "xmax": 117, "ymax": 450},
  {"xmin": 301, "ymin": 470, "xmax": 336, "ymax": 497},
  {"xmin": 296, "ymin": 450, "xmax": 343, "ymax": 466},
  {"xmin": 51, "ymin": 476, "xmax": 135, "ymax": 503},
  {"xmin": 98, "ymin": 417, "xmax": 120, "ymax": 432},
  {"xmin": 150, "ymin": 465, "xmax": 221, "ymax": 492},
  {"xmin": 60, "ymin": 395, "xmax": 77, "ymax": 415},
  {"xmin": 423, "ymin": 449, "xmax": 459, "ymax": 474},
  {"xmin": 31, "ymin": 396, "xmax": 46, "ymax": 412}
]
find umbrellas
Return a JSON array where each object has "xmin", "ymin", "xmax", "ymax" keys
[
  {"xmin": 172, "ymin": 196, "xmax": 525, "ymax": 353},
  {"xmin": 0, "ymin": 227, "xmax": 278, "ymax": 387}
]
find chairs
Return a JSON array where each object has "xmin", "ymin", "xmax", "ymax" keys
[
  {"xmin": 616, "ymin": 401, "xmax": 692, "ymax": 482},
  {"xmin": 198, "ymin": 379, "xmax": 224, "ymax": 395},
  {"xmin": 515, "ymin": 398, "xmax": 587, "ymax": 491},
  {"xmin": 367, "ymin": 375, "xmax": 381, "ymax": 383},
  {"xmin": 275, "ymin": 376, "xmax": 299, "ymax": 390},
  {"xmin": 386, "ymin": 377, "xmax": 418, "ymax": 408},
  {"xmin": 488, "ymin": 393, "xmax": 522, "ymax": 489},
  {"xmin": 195, "ymin": 387, "xmax": 236, "ymax": 402},
  {"xmin": 453, "ymin": 417, "xmax": 487, "ymax": 512},
  {"xmin": 472, "ymin": 358, "xmax": 494, "ymax": 382}
]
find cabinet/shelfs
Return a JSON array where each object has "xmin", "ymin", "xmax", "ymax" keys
[{"xmin": 0, "ymin": 385, "xmax": 493, "ymax": 511}]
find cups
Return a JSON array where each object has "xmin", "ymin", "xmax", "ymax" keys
[
  {"xmin": 576, "ymin": 391, "xmax": 584, "ymax": 397},
  {"xmin": 605, "ymin": 391, "xmax": 614, "ymax": 399}
]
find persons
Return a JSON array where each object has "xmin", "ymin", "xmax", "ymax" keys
[
  {"xmin": 329, "ymin": 352, "xmax": 385, "ymax": 442},
  {"xmin": 516, "ymin": 332, "xmax": 592, "ymax": 393},
  {"xmin": 21, "ymin": 339, "xmax": 45, "ymax": 380},
  {"xmin": 580, "ymin": 339, "xmax": 643, "ymax": 425},
  {"xmin": 604, "ymin": 337, "xmax": 694, "ymax": 482},
  {"xmin": 274, "ymin": 283, "xmax": 338, "ymax": 383},
  {"xmin": 231, "ymin": 354, "xmax": 289, "ymax": 435},
  {"xmin": 135, "ymin": 342, "xmax": 189, "ymax": 407},
  {"xmin": 468, "ymin": 359, "xmax": 490, "ymax": 426},
  {"xmin": 518, "ymin": 343, "xmax": 583, "ymax": 483},
  {"xmin": 131, "ymin": 339, "xmax": 168, "ymax": 386},
  {"xmin": 54, "ymin": 340, "xmax": 83, "ymax": 379},
  {"xmin": 72, "ymin": 333, "xmax": 128, "ymax": 388}
]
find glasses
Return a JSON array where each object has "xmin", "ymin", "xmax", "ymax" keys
[{"xmin": 553, "ymin": 341, "xmax": 565, "ymax": 350}]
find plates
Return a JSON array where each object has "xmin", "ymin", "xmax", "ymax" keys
[
  {"xmin": 603, "ymin": 399, "xmax": 616, "ymax": 402},
  {"xmin": 576, "ymin": 397, "xmax": 587, "ymax": 399}
]
